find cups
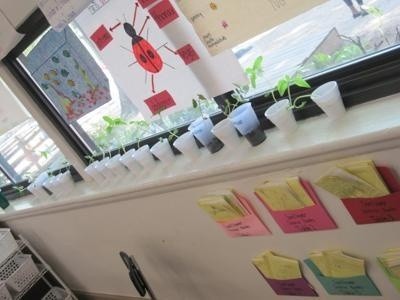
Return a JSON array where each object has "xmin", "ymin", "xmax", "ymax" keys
[
  {"xmin": 209, "ymin": 119, "xmax": 240, "ymax": 149},
  {"xmin": 227, "ymin": 103, "xmax": 267, "ymax": 146},
  {"xmin": 83, "ymin": 132, "xmax": 175, "ymax": 187},
  {"xmin": 25, "ymin": 171, "xmax": 76, "ymax": 201},
  {"xmin": 190, "ymin": 117, "xmax": 224, "ymax": 153},
  {"xmin": 310, "ymin": 80, "xmax": 346, "ymax": 123},
  {"xmin": 171, "ymin": 132, "xmax": 203, "ymax": 163},
  {"xmin": 263, "ymin": 99, "xmax": 297, "ymax": 135}
]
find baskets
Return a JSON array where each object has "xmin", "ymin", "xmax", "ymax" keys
[
  {"xmin": 0, "ymin": 280, "xmax": 12, "ymax": 300},
  {"xmin": 0, "ymin": 228, "xmax": 19, "ymax": 267},
  {"xmin": 42, "ymin": 286, "xmax": 73, "ymax": 300},
  {"xmin": 0, "ymin": 254, "xmax": 39, "ymax": 293}
]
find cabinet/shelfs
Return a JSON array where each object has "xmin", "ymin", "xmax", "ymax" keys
[{"xmin": 0, "ymin": 235, "xmax": 79, "ymax": 300}]
